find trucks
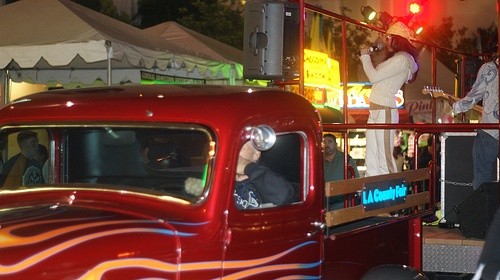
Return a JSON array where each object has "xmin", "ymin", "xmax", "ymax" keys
[{"xmin": 0, "ymin": 0, "xmax": 498, "ymax": 280}]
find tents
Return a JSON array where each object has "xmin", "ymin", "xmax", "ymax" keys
[{"xmin": 0, "ymin": 1, "xmax": 263, "ymax": 89}]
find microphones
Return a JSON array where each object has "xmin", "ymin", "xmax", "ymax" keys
[{"xmin": 355, "ymin": 44, "xmax": 384, "ymax": 58}]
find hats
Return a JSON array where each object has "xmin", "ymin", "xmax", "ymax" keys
[{"xmin": 384, "ymin": 21, "xmax": 415, "ymax": 42}]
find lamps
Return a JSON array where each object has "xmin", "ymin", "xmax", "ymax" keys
[
  {"xmin": 411, "ymin": 21, "xmax": 423, "ymax": 35},
  {"xmin": 361, "ymin": 6, "xmax": 377, "ymax": 21}
]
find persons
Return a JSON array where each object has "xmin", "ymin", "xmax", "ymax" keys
[
  {"xmin": 358, "ymin": 23, "xmax": 418, "ymax": 178},
  {"xmin": 323, "ymin": 134, "xmax": 359, "ymax": 211},
  {"xmin": 441, "ymin": 50, "xmax": 499, "ymax": 188},
  {"xmin": 234, "ymin": 141, "xmax": 294, "ymax": 210},
  {"xmin": 392, "ymin": 137, "xmax": 444, "ymax": 209},
  {"xmin": 0, "ymin": 131, "xmax": 51, "ymax": 188}
]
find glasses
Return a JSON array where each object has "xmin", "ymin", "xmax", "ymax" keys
[{"xmin": 384, "ymin": 35, "xmax": 399, "ymax": 42}]
